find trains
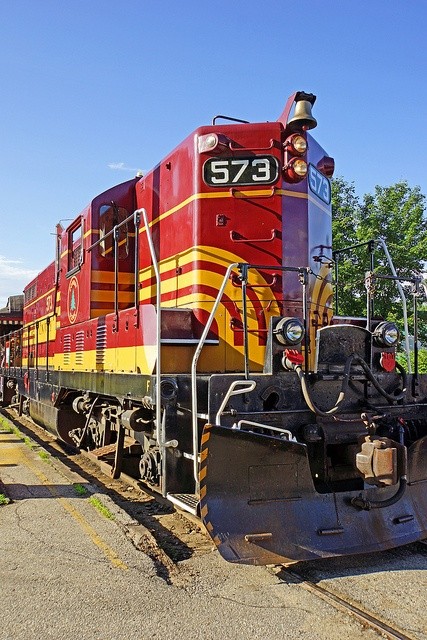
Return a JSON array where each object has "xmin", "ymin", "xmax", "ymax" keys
[{"xmin": 1, "ymin": 89, "xmax": 424, "ymax": 571}]
